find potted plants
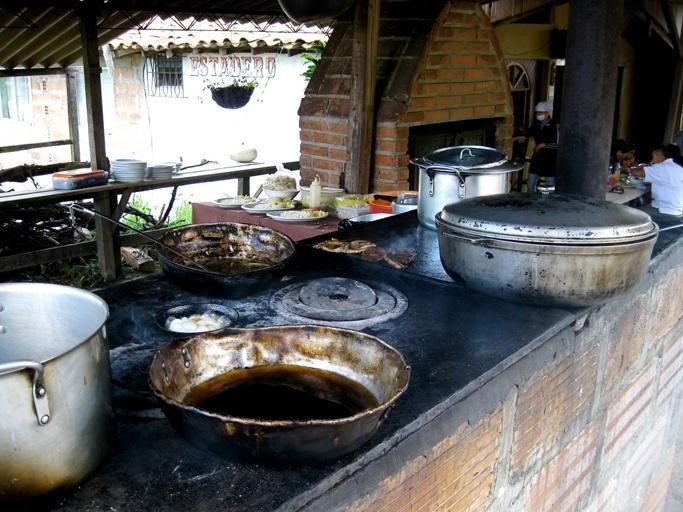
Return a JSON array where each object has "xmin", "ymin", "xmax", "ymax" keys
[{"xmin": 201, "ymin": 71, "xmax": 258, "ymax": 109}]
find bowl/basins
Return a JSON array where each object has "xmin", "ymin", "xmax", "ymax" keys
[
  {"xmin": 263, "ymin": 187, "xmax": 300, "ymax": 201},
  {"xmin": 156, "ymin": 222, "xmax": 297, "ymax": 293},
  {"xmin": 147, "ymin": 324, "xmax": 412, "ymax": 467}
]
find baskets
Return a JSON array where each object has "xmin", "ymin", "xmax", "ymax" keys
[{"xmin": 212, "ymin": 83, "xmax": 254, "ymax": 109}]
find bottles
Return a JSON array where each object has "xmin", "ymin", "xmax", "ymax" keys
[{"xmin": 309, "ymin": 174, "xmax": 322, "ymax": 211}]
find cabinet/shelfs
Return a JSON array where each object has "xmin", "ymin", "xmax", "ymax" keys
[{"xmin": 0, "ymin": 158, "xmax": 300, "ymax": 282}]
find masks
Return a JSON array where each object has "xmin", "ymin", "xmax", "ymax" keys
[{"xmin": 537, "ymin": 115, "xmax": 545, "ymax": 120}]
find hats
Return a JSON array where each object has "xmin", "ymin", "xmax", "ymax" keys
[{"xmin": 535, "ymin": 102, "xmax": 553, "ymax": 112}]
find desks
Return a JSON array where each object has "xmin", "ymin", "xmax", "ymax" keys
[{"xmin": 604, "ymin": 180, "xmax": 653, "ymax": 208}]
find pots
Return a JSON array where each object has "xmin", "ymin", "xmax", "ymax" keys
[
  {"xmin": 409, "ymin": 144, "xmax": 526, "ymax": 232},
  {"xmin": 153, "ymin": 302, "xmax": 240, "ymax": 338},
  {"xmin": 300, "ymin": 185, "xmax": 345, "ymax": 209},
  {"xmin": 434, "ymin": 182, "xmax": 660, "ymax": 308},
  {"xmin": 374, "ymin": 192, "xmax": 418, "ymax": 216},
  {"xmin": 1, "ymin": 282, "xmax": 113, "ymax": 499}
]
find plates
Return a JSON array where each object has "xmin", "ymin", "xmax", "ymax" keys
[
  {"xmin": 240, "ymin": 200, "xmax": 295, "ymax": 214},
  {"xmin": 212, "ymin": 196, "xmax": 245, "ymax": 209},
  {"xmin": 265, "ymin": 209, "xmax": 329, "ymax": 223},
  {"xmin": 110, "ymin": 158, "xmax": 147, "ymax": 183},
  {"xmin": 147, "ymin": 165, "xmax": 174, "ymax": 181}
]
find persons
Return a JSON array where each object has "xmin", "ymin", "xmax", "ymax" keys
[
  {"xmin": 630, "ymin": 143, "xmax": 682, "ymax": 226},
  {"xmin": 608, "ymin": 139, "xmax": 629, "ymax": 176},
  {"xmin": 518, "ymin": 101, "xmax": 556, "ymax": 194},
  {"xmin": 620, "ymin": 145, "xmax": 635, "ymax": 168}
]
